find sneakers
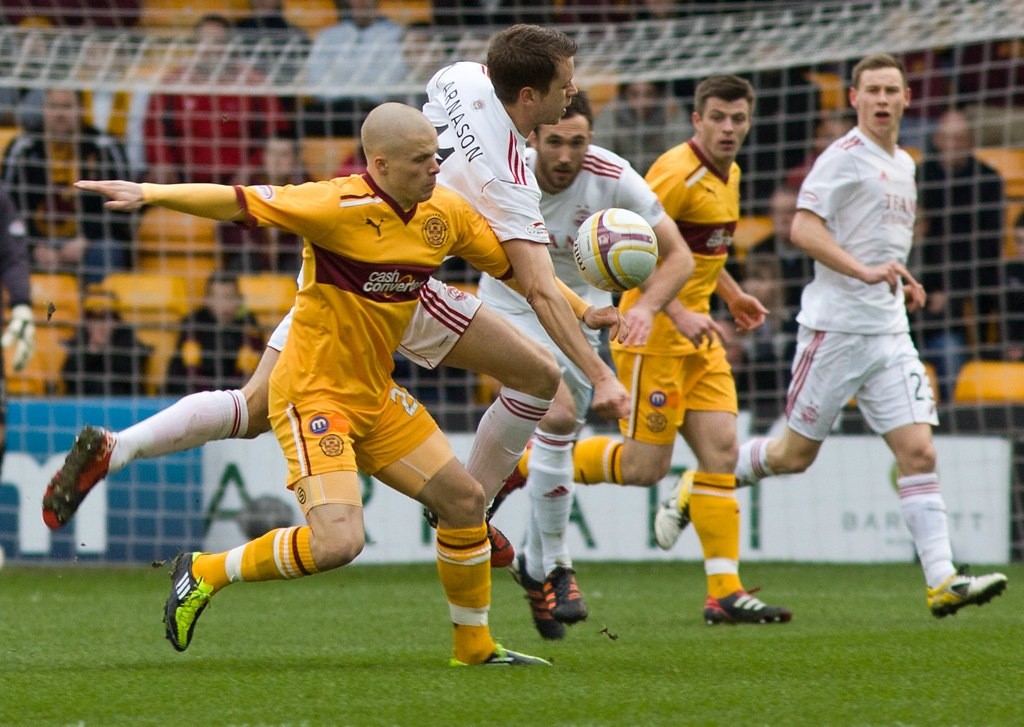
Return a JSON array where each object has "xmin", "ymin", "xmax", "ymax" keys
[
  {"xmin": 450, "ymin": 643, "xmax": 554, "ymax": 667},
  {"xmin": 926, "ymin": 563, "xmax": 1008, "ymax": 619},
  {"xmin": 508, "ymin": 553, "xmax": 566, "ymax": 640},
  {"xmin": 703, "ymin": 587, "xmax": 792, "ymax": 625},
  {"xmin": 163, "ymin": 551, "xmax": 214, "ymax": 651},
  {"xmin": 42, "ymin": 425, "xmax": 117, "ymax": 532},
  {"xmin": 424, "ymin": 507, "xmax": 515, "ymax": 568},
  {"xmin": 654, "ymin": 470, "xmax": 695, "ymax": 551},
  {"xmin": 542, "ymin": 559, "xmax": 589, "ymax": 626}
]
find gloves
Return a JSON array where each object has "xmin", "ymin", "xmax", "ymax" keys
[{"xmin": 1, "ymin": 305, "xmax": 35, "ymax": 372}]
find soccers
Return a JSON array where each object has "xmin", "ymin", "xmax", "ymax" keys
[{"xmin": 572, "ymin": 207, "xmax": 660, "ymax": 293}]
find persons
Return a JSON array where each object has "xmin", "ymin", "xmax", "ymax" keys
[
  {"xmin": 388, "ymin": 0, "xmax": 1022, "ymax": 434},
  {"xmin": 651, "ymin": 53, "xmax": 1007, "ymax": 618},
  {"xmin": 41, "ymin": 22, "xmax": 636, "ymax": 566},
  {"xmin": 488, "ymin": 74, "xmax": 797, "ymax": 628},
  {"xmin": 476, "ymin": 86, "xmax": 694, "ymax": 639},
  {"xmin": 71, "ymin": 101, "xmax": 623, "ymax": 667},
  {"xmin": 0, "ymin": 0, "xmax": 407, "ymax": 399}
]
